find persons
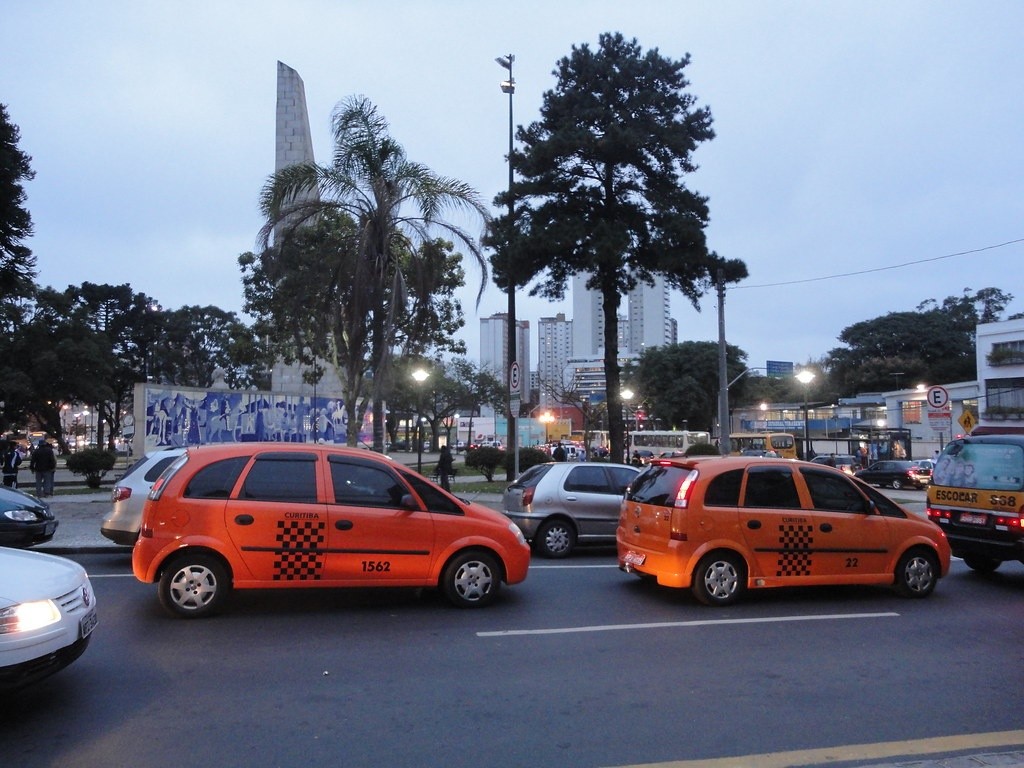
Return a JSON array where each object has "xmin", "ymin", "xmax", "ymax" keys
[
  {"xmin": 0, "ymin": 441, "xmax": 23, "ymax": 490},
  {"xmin": 439, "ymin": 445, "xmax": 454, "ymax": 494},
  {"xmin": 553, "ymin": 443, "xmax": 565, "ymax": 462},
  {"xmin": 827, "ymin": 453, "xmax": 836, "ymax": 468},
  {"xmin": 934, "ymin": 458, "xmax": 977, "ymax": 488},
  {"xmin": 631, "ymin": 449, "xmax": 644, "ymax": 467},
  {"xmin": 29, "ymin": 438, "xmax": 58, "ymax": 498},
  {"xmin": 579, "ymin": 447, "xmax": 607, "ymax": 462}
]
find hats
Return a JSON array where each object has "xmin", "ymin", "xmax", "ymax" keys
[{"xmin": 38, "ymin": 440, "xmax": 47, "ymax": 445}]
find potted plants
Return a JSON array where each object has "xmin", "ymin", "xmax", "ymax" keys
[{"xmin": 66, "ymin": 448, "xmax": 116, "ymax": 489}]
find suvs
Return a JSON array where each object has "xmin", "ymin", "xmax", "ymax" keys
[
  {"xmin": 855, "ymin": 458, "xmax": 937, "ymax": 490},
  {"xmin": 810, "ymin": 453, "xmax": 858, "ymax": 475},
  {"xmin": 926, "ymin": 424, "xmax": 1024, "ymax": 573}
]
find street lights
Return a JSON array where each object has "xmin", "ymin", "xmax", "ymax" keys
[
  {"xmin": 795, "ymin": 370, "xmax": 815, "ymax": 462},
  {"xmin": 82, "ymin": 410, "xmax": 90, "ymax": 451},
  {"xmin": 411, "ymin": 368, "xmax": 430, "ymax": 472},
  {"xmin": 620, "ymin": 388, "xmax": 635, "ymax": 465},
  {"xmin": 541, "ymin": 412, "xmax": 555, "ymax": 443},
  {"xmin": 454, "ymin": 414, "xmax": 460, "ymax": 455},
  {"xmin": 73, "ymin": 411, "xmax": 81, "ymax": 453},
  {"xmin": 496, "ymin": 53, "xmax": 516, "ymax": 475},
  {"xmin": 61, "ymin": 404, "xmax": 68, "ymax": 442}
]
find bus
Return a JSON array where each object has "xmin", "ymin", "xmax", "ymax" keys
[
  {"xmin": 571, "ymin": 430, "xmax": 610, "ymax": 453},
  {"xmin": 716, "ymin": 433, "xmax": 798, "ymax": 459},
  {"xmin": 622, "ymin": 429, "xmax": 711, "ymax": 459}
]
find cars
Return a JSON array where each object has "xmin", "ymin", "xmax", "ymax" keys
[
  {"xmin": 0, "ymin": 545, "xmax": 100, "ymax": 696},
  {"xmin": 100, "ymin": 442, "xmax": 194, "ymax": 546},
  {"xmin": 616, "ymin": 454, "xmax": 951, "ymax": 610},
  {"xmin": 0, "ymin": 483, "xmax": 60, "ymax": 550},
  {"xmin": 385, "ymin": 437, "xmax": 502, "ymax": 454},
  {"xmin": 533, "ymin": 437, "xmax": 610, "ymax": 462},
  {"xmin": 0, "ymin": 430, "xmax": 131, "ymax": 460},
  {"xmin": 132, "ymin": 440, "xmax": 532, "ymax": 620},
  {"xmin": 500, "ymin": 461, "xmax": 646, "ymax": 560}
]
